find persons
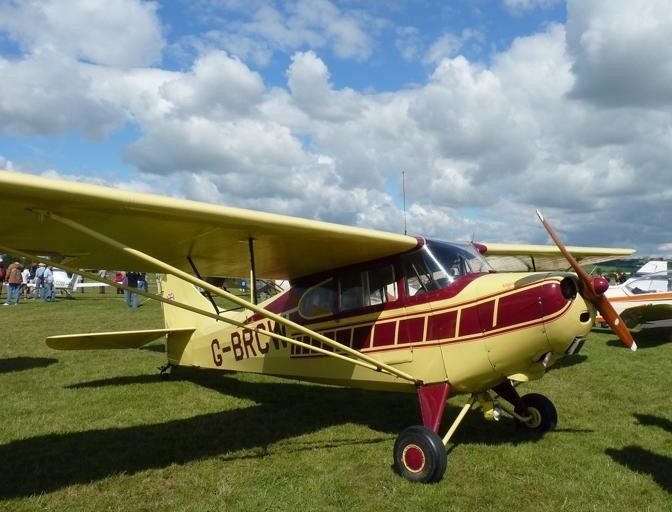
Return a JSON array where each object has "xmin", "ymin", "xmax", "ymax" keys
[
  {"xmin": 600, "ymin": 270, "xmax": 638, "ymax": 286},
  {"xmin": 0, "ymin": 257, "xmax": 162, "ymax": 309}
]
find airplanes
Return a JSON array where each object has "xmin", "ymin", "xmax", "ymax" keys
[
  {"xmin": 595, "ymin": 260, "xmax": 672, "ymax": 333},
  {"xmin": 1, "ymin": 266, "xmax": 139, "ymax": 299},
  {"xmin": 0, "ymin": 169, "xmax": 638, "ymax": 484}
]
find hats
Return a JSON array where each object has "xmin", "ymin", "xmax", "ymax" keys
[{"xmin": 38, "ymin": 262, "xmax": 45, "ymax": 266}]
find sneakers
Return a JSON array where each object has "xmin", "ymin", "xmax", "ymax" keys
[{"xmin": 2, "ymin": 302, "xmax": 9, "ymax": 306}]
